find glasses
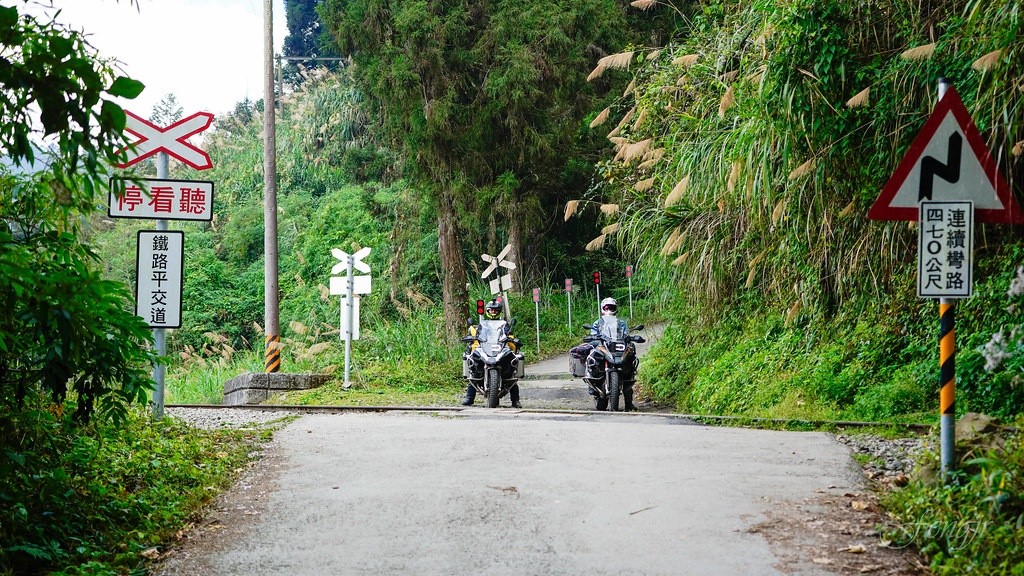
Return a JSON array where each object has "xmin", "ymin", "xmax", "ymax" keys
[{"xmin": 603, "ymin": 305, "xmax": 616, "ymax": 311}]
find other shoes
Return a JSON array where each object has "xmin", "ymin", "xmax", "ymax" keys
[
  {"xmin": 625, "ymin": 404, "xmax": 637, "ymax": 412},
  {"xmin": 461, "ymin": 398, "xmax": 474, "ymax": 405},
  {"xmin": 589, "ymin": 381, "xmax": 596, "ymax": 395},
  {"xmin": 512, "ymin": 400, "xmax": 522, "ymax": 408}
]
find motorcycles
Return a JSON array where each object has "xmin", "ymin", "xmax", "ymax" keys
[
  {"xmin": 463, "ymin": 318, "xmax": 521, "ymax": 408},
  {"xmin": 581, "ymin": 323, "xmax": 647, "ymax": 413}
]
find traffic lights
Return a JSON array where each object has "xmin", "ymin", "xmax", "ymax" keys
[
  {"xmin": 476, "ymin": 299, "xmax": 485, "ymax": 314},
  {"xmin": 565, "ymin": 279, "xmax": 572, "ymax": 291},
  {"xmin": 594, "ymin": 271, "xmax": 602, "ymax": 285},
  {"xmin": 533, "ymin": 288, "xmax": 540, "ymax": 303},
  {"xmin": 626, "ymin": 265, "xmax": 633, "ymax": 278}
]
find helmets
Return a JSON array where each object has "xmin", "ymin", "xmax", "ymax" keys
[
  {"xmin": 486, "ymin": 299, "xmax": 503, "ymax": 320},
  {"xmin": 601, "ymin": 297, "xmax": 618, "ymax": 316}
]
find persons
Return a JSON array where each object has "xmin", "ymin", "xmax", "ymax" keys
[
  {"xmin": 588, "ymin": 297, "xmax": 637, "ymax": 412},
  {"xmin": 462, "ymin": 301, "xmax": 522, "ymax": 408}
]
